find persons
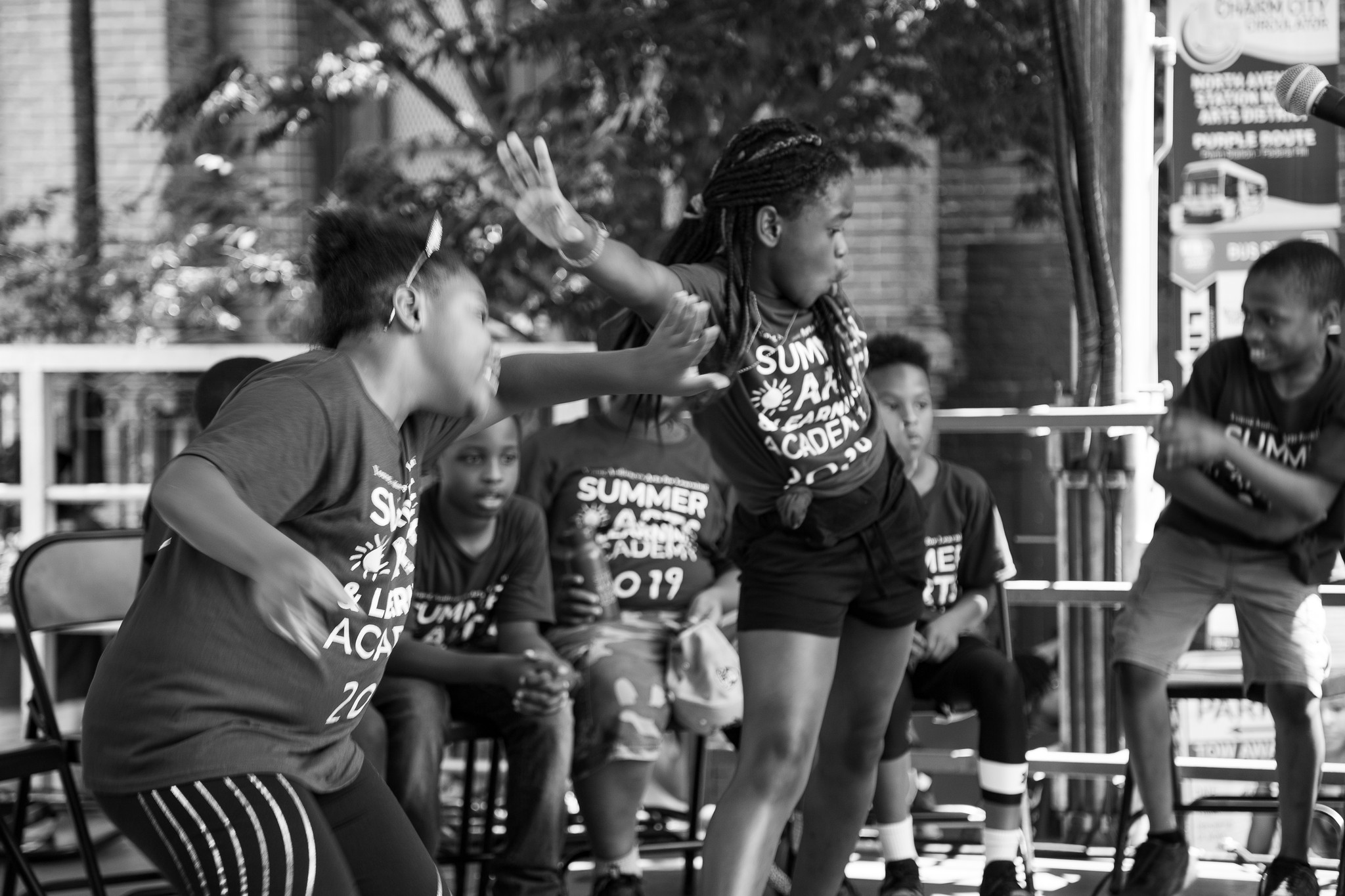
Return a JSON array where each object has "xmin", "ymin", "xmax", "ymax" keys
[
  {"xmin": 1110, "ymin": 239, "xmax": 1345, "ymax": 896},
  {"xmin": 479, "ymin": 117, "xmax": 930, "ymax": 896},
  {"xmin": 79, "ymin": 208, "xmax": 734, "ymax": 896},
  {"xmin": 137, "ymin": 308, "xmax": 1035, "ymax": 896}
]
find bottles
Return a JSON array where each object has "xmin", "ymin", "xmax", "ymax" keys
[{"xmin": 571, "ymin": 525, "xmax": 619, "ymax": 623}]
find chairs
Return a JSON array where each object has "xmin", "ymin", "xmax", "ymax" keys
[
  {"xmin": 0, "ymin": 529, "xmax": 1036, "ymax": 896},
  {"xmin": 1102, "ymin": 649, "xmax": 1345, "ymax": 896}
]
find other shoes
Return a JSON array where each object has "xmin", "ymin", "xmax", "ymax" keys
[
  {"xmin": 1122, "ymin": 838, "xmax": 1189, "ymax": 896},
  {"xmin": 1257, "ymin": 864, "xmax": 1319, "ymax": 896},
  {"xmin": 769, "ymin": 848, "xmax": 860, "ymax": 896}
]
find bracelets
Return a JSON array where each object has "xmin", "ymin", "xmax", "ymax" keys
[{"xmin": 558, "ymin": 213, "xmax": 610, "ymax": 268}]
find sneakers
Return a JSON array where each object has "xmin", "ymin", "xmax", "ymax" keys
[
  {"xmin": 980, "ymin": 860, "xmax": 1035, "ymax": 896},
  {"xmin": 877, "ymin": 858, "xmax": 925, "ymax": 896},
  {"xmin": 589, "ymin": 866, "xmax": 646, "ymax": 896}
]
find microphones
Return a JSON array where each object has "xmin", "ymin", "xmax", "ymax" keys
[{"xmin": 1275, "ymin": 63, "xmax": 1345, "ymax": 130}]
